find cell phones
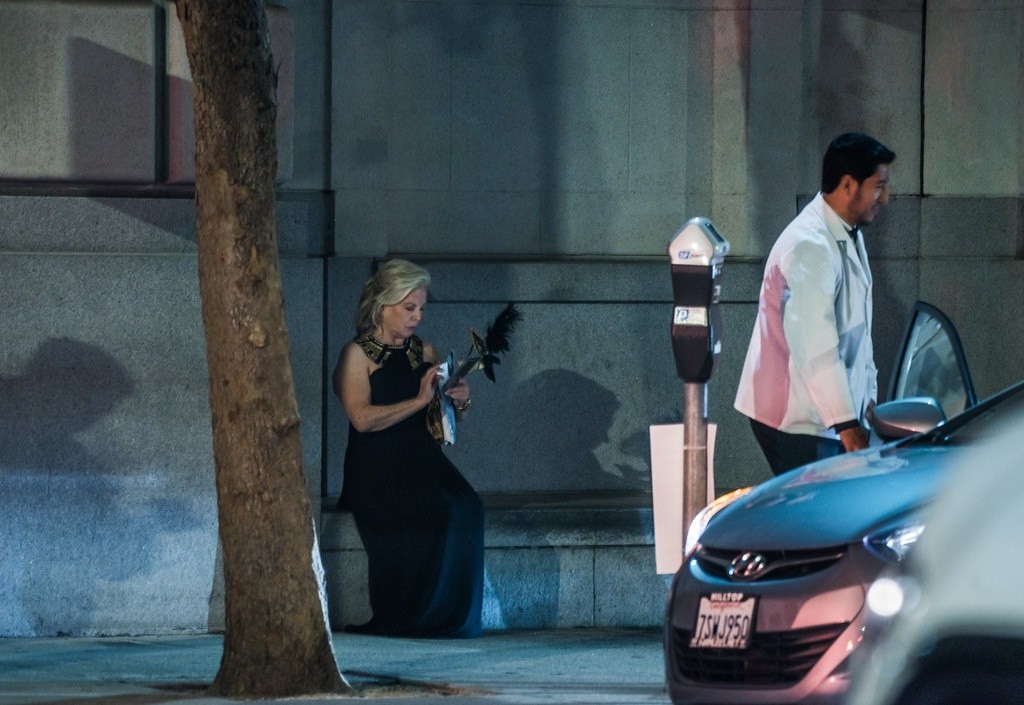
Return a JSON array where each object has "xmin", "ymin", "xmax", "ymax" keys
[{"xmin": 448, "ymin": 349, "xmax": 456, "ymax": 376}]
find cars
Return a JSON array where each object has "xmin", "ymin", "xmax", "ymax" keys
[{"xmin": 658, "ymin": 299, "xmax": 1024, "ymax": 705}]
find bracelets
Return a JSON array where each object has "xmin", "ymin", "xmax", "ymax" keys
[{"xmin": 457, "ymin": 400, "xmax": 472, "ymax": 412}]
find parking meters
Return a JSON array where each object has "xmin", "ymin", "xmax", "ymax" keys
[{"xmin": 668, "ymin": 217, "xmax": 729, "ymax": 557}]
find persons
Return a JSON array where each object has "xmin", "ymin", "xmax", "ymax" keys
[
  {"xmin": 733, "ymin": 130, "xmax": 897, "ymax": 478},
  {"xmin": 332, "ymin": 261, "xmax": 486, "ymax": 639}
]
